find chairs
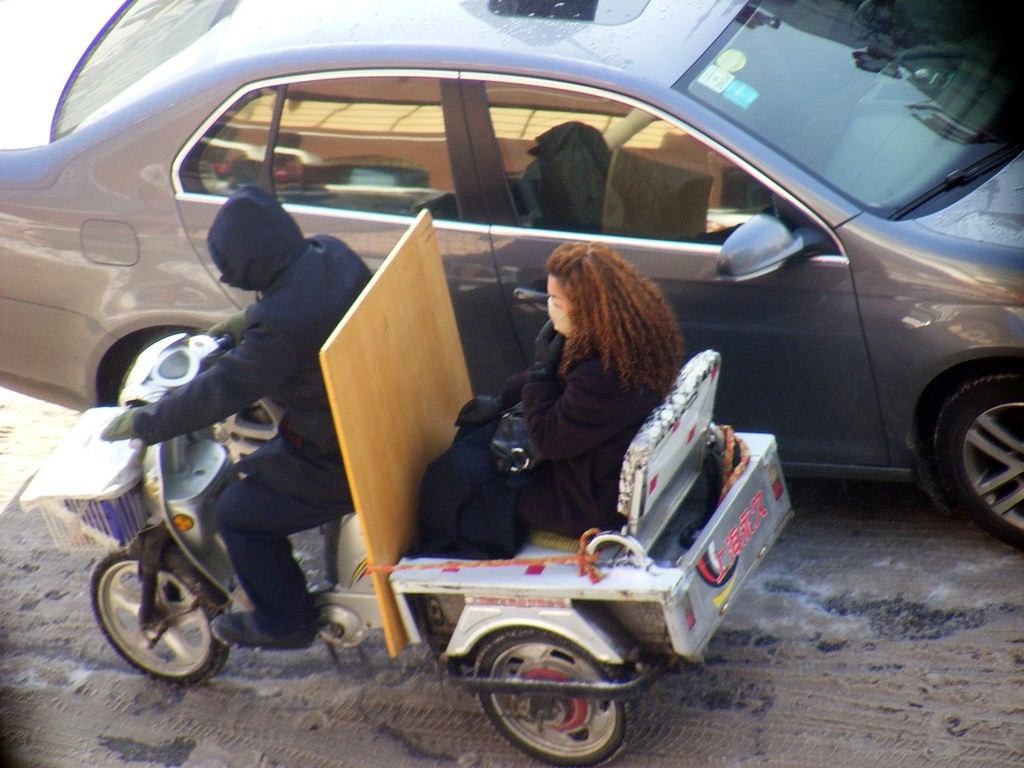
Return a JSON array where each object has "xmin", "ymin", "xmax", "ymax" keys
[{"xmin": 512, "ymin": 349, "xmax": 723, "ymax": 572}]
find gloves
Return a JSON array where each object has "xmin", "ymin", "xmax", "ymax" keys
[
  {"xmin": 454, "ymin": 395, "xmax": 503, "ymax": 426},
  {"xmin": 526, "ymin": 318, "xmax": 565, "ymax": 382},
  {"xmin": 205, "ymin": 309, "xmax": 248, "ymax": 349},
  {"xmin": 99, "ymin": 407, "xmax": 139, "ymax": 442}
]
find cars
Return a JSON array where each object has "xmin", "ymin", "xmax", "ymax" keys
[
  {"xmin": 214, "ymin": 152, "xmax": 301, "ymax": 189},
  {"xmin": 0, "ymin": 1, "xmax": 1024, "ymax": 549}
]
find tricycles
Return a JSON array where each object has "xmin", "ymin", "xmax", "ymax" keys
[{"xmin": 18, "ymin": 332, "xmax": 797, "ymax": 768}]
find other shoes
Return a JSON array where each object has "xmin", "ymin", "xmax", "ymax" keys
[{"xmin": 210, "ymin": 611, "xmax": 318, "ymax": 653}]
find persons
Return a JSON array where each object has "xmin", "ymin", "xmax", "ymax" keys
[
  {"xmin": 414, "ymin": 122, "xmax": 613, "ymax": 233},
  {"xmin": 100, "ymin": 186, "xmax": 374, "ymax": 650},
  {"xmin": 414, "ymin": 241, "xmax": 683, "ymax": 558}
]
